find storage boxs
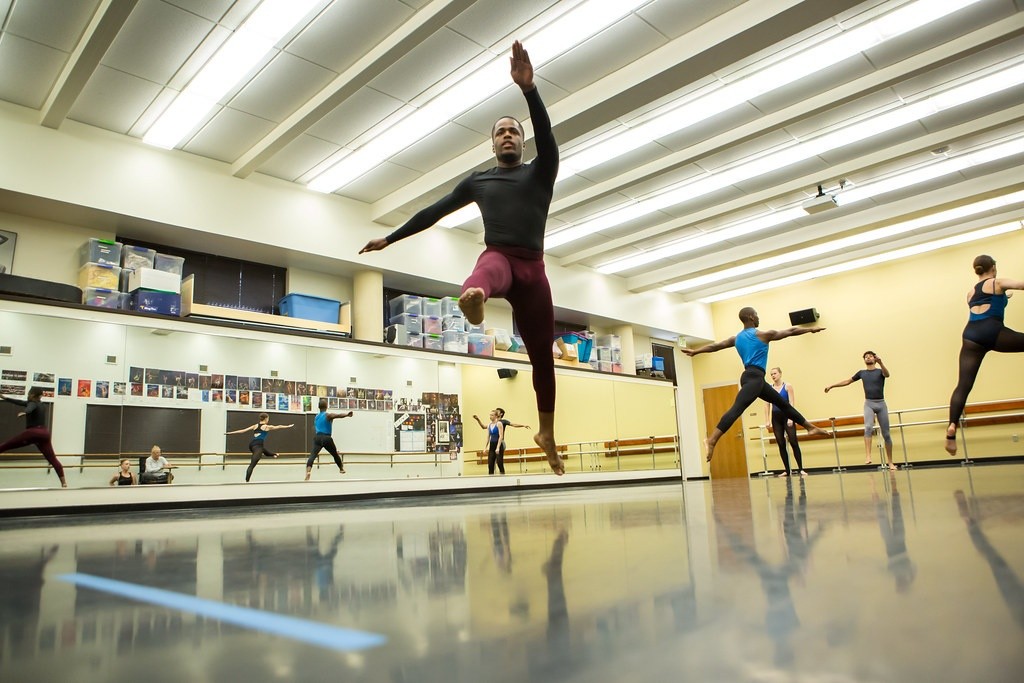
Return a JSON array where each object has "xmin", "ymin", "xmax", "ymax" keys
[
  {"xmin": 76, "ymin": 238, "xmax": 185, "ymax": 317},
  {"xmin": 495, "ymin": 333, "xmax": 527, "ymax": 354},
  {"xmin": 278, "ymin": 293, "xmax": 340, "ymax": 323},
  {"xmin": 652, "ymin": 355, "xmax": 665, "ymax": 371},
  {"xmin": 553, "ymin": 330, "xmax": 622, "ymax": 374},
  {"xmin": 389, "ymin": 294, "xmax": 495, "ymax": 358}
]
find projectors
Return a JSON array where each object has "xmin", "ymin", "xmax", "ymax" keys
[{"xmin": 803, "ymin": 195, "xmax": 839, "ymax": 215}]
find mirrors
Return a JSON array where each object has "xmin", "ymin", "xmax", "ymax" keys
[{"xmin": 0, "ymin": 310, "xmax": 682, "ymax": 492}]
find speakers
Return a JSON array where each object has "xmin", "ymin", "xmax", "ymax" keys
[
  {"xmin": 789, "ymin": 308, "xmax": 819, "ymax": 326},
  {"xmin": 497, "ymin": 368, "xmax": 518, "ymax": 378}
]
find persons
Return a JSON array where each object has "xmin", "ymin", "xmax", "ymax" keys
[
  {"xmin": 824, "ymin": 350, "xmax": 898, "ymax": 470},
  {"xmin": 359, "ymin": 39, "xmax": 565, "ymax": 477},
  {"xmin": 142, "ymin": 446, "xmax": 174, "ymax": 485},
  {"xmin": 680, "ymin": 307, "xmax": 831, "ymax": 462},
  {"xmin": 944, "ymin": 255, "xmax": 1024, "ymax": 456},
  {"xmin": 305, "ymin": 400, "xmax": 353, "ymax": 481},
  {"xmin": 224, "ymin": 413, "xmax": 294, "ymax": 482},
  {"xmin": 765, "ymin": 367, "xmax": 809, "ymax": 477},
  {"xmin": 473, "ymin": 408, "xmax": 531, "ymax": 474},
  {"xmin": 0, "ymin": 386, "xmax": 67, "ymax": 487},
  {"xmin": 109, "ymin": 460, "xmax": 138, "ymax": 486},
  {"xmin": 483, "ymin": 410, "xmax": 506, "ymax": 474}
]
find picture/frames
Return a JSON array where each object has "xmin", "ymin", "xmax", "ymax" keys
[{"xmin": 0, "ymin": 228, "xmax": 18, "ymax": 275}]
